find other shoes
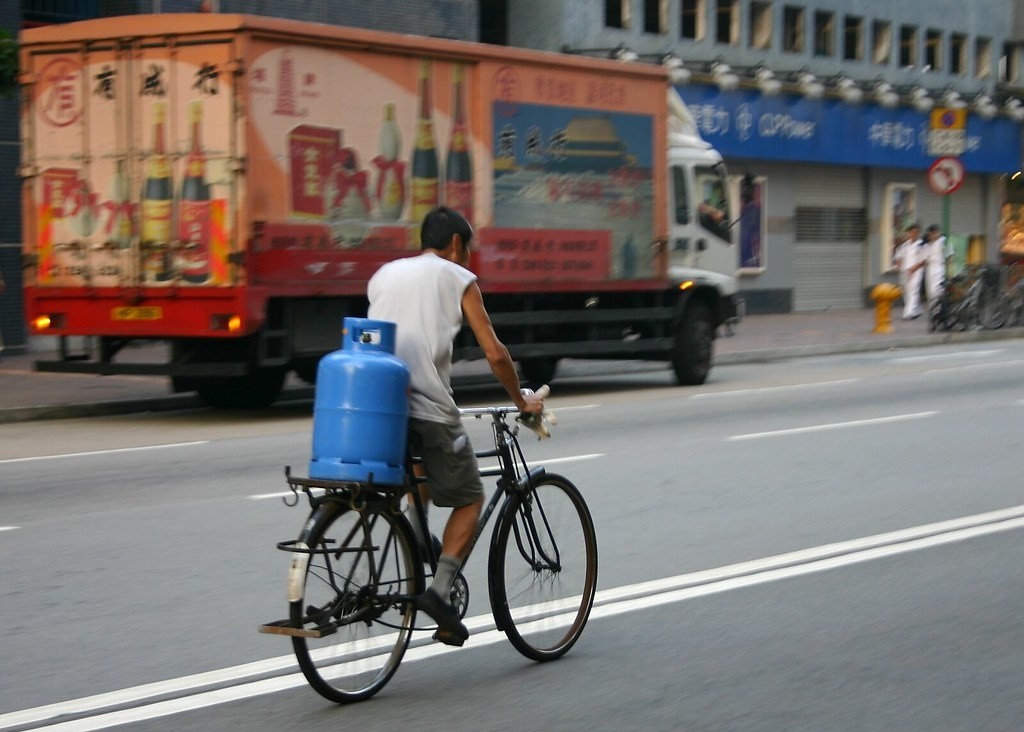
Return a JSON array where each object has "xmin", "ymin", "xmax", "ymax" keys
[
  {"xmin": 419, "ymin": 534, "xmax": 444, "ymax": 563},
  {"xmin": 418, "ymin": 588, "xmax": 470, "ymax": 642}
]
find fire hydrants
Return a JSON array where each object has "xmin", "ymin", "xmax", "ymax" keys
[{"xmin": 869, "ymin": 282, "xmax": 901, "ymax": 337}]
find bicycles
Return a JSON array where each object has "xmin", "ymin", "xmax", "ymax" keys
[
  {"xmin": 257, "ymin": 396, "xmax": 600, "ymax": 702},
  {"xmin": 926, "ymin": 265, "xmax": 1021, "ymax": 338}
]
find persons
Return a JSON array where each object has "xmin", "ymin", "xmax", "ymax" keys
[
  {"xmin": 676, "ymin": 202, "xmax": 725, "ymax": 235},
  {"xmin": 366, "ymin": 206, "xmax": 543, "ymax": 641},
  {"xmin": 892, "ymin": 223, "xmax": 955, "ymax": 320}
]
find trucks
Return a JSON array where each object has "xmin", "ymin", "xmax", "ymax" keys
[{"xmin": 17, "ymin": 11, "xmax": 759, "ymax": 401}]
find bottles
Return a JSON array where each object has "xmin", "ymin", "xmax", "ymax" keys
[
  {"xmin": 326, "ymin": 150, "xmax": 369, "ymax": 248},
  {"xmin": 444, "ymin": 62, "xmax": 474, "ymax": 228},
  {"xmin": 64, "ymin": 171, "xmax": 91, "ymax": 241},
  {"xmin": 410, "ymin": 58, "xmax": 440, "ymax": 248},
  {"xmin": 179, "ymin": 101, "xmax": 209, "ymax": 283},
  {"xmin": 95, "ymin": 159, "xmax": 131, "ymax": 247},
  {"xmin": 138, "ymin": 102, "xmax": 174, "ymax": 282},
  {"xmin": 370, "ymin": 103, "xmax": 405, "ymax": 220}
]
